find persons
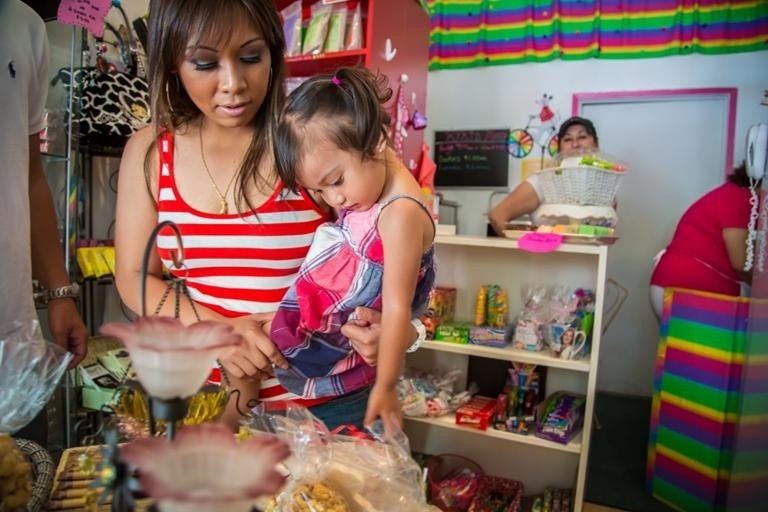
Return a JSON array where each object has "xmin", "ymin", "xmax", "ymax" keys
[
  {"xmin": 0, "ymin": 0, "xmax": 88, "ymax": 452},
  {"xmin": 555, "ymin": 328, "xmax": 574, "ymax": 359},
  {"xmin": 221, "ymin": 66, "xmax": 436, "ymax": 442},
  {"xmin": 490, "ymin": 116, "xmax": 618, "ymax": 238},
  {"xmin": 648, "ymin": 158, "xmax": 758, "ymax": 327},
  {"xmin": 116, "ymin": 1, "xmax": 428, "ymax": 446}
]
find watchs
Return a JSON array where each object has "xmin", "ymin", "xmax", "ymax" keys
[
  {"xmin": 44, "ymin": 282, "xmax": 80, "ymax": 302},
  {"xmin": 406, "ymin": 318, "xmax": 427, "ymax": 353}
]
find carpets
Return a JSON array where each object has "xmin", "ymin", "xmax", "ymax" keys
[{"xmin": 581, "ymin": 390, "xmax": 675, "ymax": 511}]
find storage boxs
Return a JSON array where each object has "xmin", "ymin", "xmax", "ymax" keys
[{"xmin": 532, "ymin": 165, "xmax": 624, "ymax": 208}]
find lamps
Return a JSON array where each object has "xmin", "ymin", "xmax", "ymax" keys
[{"xmin": 98, "ymin": 225, "xmax": 293, "ymax": 511}]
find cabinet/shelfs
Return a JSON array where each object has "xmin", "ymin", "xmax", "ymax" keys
[
  {"xmin": 397, "ymin": 234, "xmax": 610, "ymax": 510},
  {"xmin": 268, "ymin": 1, "xmax": 431, "ymax": 194},
  {"xmin": 44, "ymin": 1, "xmax": 137, "ymax": 346}
]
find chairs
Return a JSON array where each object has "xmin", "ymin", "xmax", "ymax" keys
[{"xmin": 592, "ymin": 279, "xmax": 631, "ymax": 432}]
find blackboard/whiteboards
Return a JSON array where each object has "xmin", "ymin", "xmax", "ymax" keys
[{"xmin": 431, "ymin": 128, "xmax": 512, "ymax": 195}]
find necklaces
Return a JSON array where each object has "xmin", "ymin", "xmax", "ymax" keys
[{"xmin": 199, "ymin": 113, "xmax": 253, "ymax": 216}]
